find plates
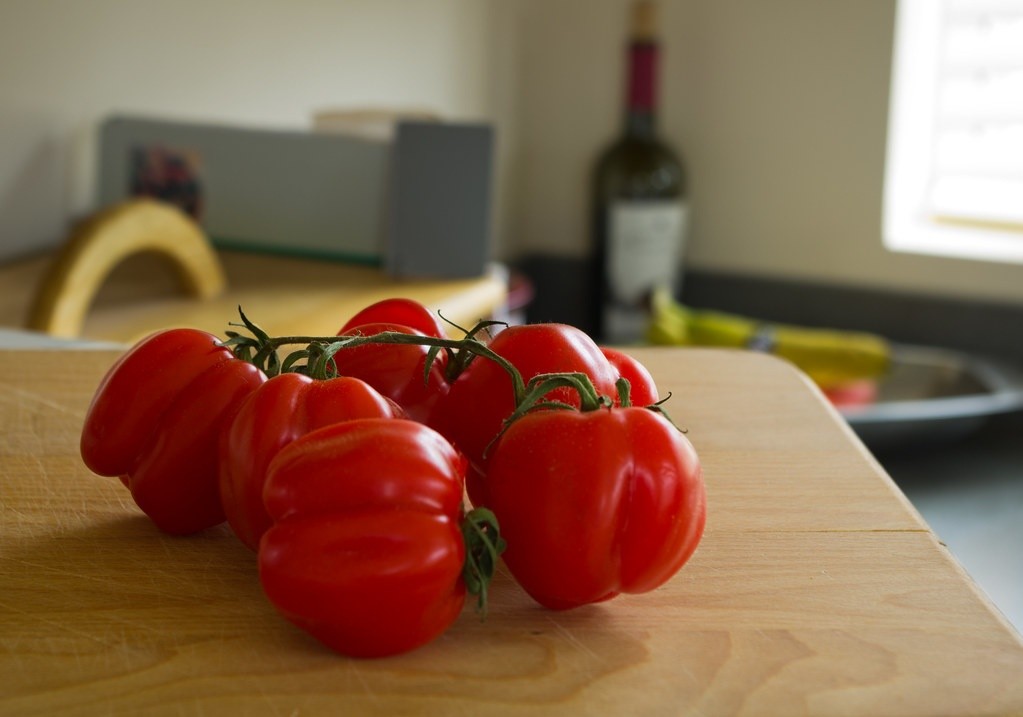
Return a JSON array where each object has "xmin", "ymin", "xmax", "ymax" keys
[{"xmin": 837, "ymin": 349, "xmax": 1023, "ymax": 442}]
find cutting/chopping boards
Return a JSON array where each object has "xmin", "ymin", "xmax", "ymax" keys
[{"xmin": 2, "ymin": 346, "xmax": 1023, "ymax": 715}]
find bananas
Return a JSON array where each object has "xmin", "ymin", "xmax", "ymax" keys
[{"xmin": 643, "ymin": 282, "xmax": 904, "ymax": 391}]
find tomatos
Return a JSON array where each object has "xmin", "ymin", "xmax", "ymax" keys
[{"xmin": 79, "ymin": 297, "xmax": 709, "ymax": 659}]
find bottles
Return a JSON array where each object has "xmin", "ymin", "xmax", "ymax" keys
[{"xmin": 592, "ymin": 0, "xmax": 689, "ymax": 346}]
